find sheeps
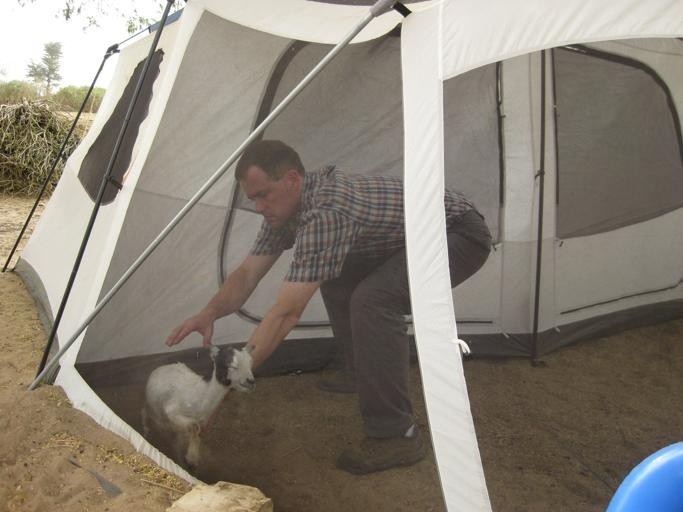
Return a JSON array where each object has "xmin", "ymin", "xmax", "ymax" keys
[{"xmin": 139, "ymin": 340, "xmax": 258, "ymax": 468}]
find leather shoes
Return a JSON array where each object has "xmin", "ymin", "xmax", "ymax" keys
[
  {"xmin": 338, "ymin": 425, "xmax": 427, "ymax": 475},
  {"xmin": 318, "ymin": 366, "xmax": 355, "ymax": 394}
]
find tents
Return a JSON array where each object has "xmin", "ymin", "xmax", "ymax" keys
[{"xmin": 2, "ymin": 0, "xmax": 683, "ymax": 512}]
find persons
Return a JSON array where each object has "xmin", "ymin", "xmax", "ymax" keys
[{"xmin": 165, "ymin": 140, "xmax": 492, "ymax": 475}]
